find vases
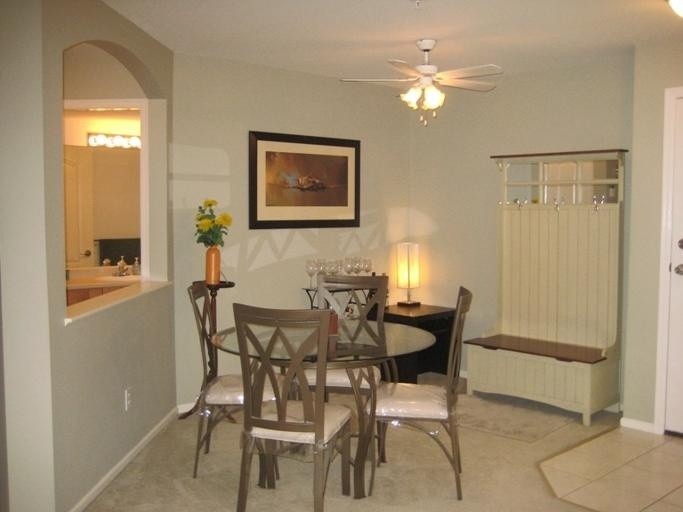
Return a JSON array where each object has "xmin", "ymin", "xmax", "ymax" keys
[{"xmin": 206, "ymin": 244, "xmax": 220, "ymax": 285}]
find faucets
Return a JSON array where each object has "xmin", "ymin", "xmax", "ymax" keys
[{"xmin": 117, "ymin": 264, "xmax": 129, "ymax": 276}]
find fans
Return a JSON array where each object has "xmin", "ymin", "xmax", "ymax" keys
[{"xmin": 342, "ymin": 38, "xmax": 504, "ymax": 127}]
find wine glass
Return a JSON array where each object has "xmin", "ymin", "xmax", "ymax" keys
[{"xmin": 306, "ymin": 256, "xmax": 372, "ymax": 289}]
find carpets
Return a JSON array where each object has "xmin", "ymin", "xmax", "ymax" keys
[{"xmin": 456, "ymin": 391, "xmax": 582, "ymax": 443}]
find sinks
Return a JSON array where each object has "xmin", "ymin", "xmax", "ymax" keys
[{"xmin": 94, "ymin": 273, "xmax": 141, "ymax": 282}]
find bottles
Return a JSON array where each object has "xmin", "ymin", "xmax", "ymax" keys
[
  {"xmin": 118, "ymin": 255, "xmax": 127, "ymax": 272},
  {"xmin": 133, "ymin": 256, "xmax": 141, "ymax": 275}
]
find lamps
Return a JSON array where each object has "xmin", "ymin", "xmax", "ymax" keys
[
  {"xmin": 397, "ymin": 76, "xmax": 446, "ymax": 127},
  {"xmin": 396, "ymin": 242, "xmax": 421, "ymax": 308}
]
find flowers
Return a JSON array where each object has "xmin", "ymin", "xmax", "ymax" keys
[{"xmin": 194, "ymin": 199, "xmax": 233, "ymax": 247}]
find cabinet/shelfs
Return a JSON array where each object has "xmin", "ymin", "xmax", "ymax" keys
[{"xmin": 462, "ymin": 146, "xmax": 627, "ymax": 426}]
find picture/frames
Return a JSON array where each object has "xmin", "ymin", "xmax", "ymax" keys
[{"xmin": 247, "ymin": 130, "xmax": 360, "ymax": 229}]
